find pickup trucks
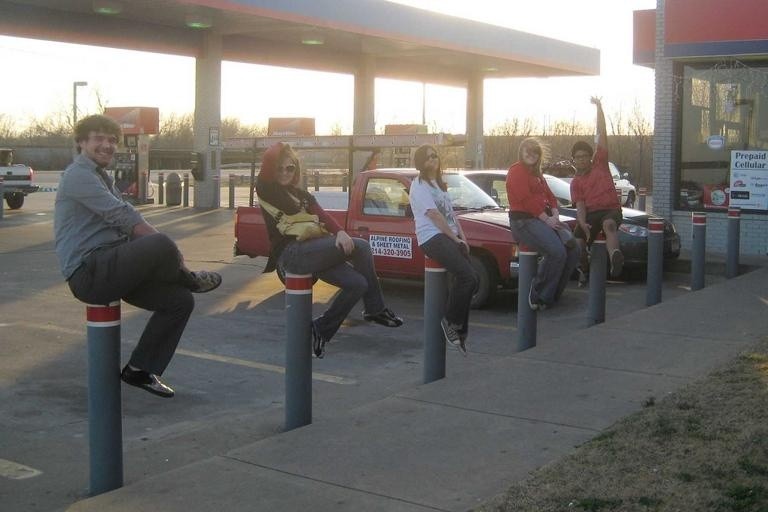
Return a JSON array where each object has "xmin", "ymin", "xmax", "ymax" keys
[
  {"xmin": 233, "ymin": 167, "xmax": 580, "ymax": 310},
  {"xmin": 0, "ymin": 145, "xmax": 40, "ymax": 209}
]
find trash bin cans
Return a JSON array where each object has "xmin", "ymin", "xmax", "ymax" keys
[{"xmin": 166, "ymin": 172, "xmax": 181, "ymax": 205}]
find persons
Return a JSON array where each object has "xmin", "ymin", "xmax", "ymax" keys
[
  {"xmin": 506, "ymin": 138, "xmax": 581, "ymax": 311},
  {"xmin": 570, "ymin": 96, "xmax": 624, "ymax": 288},
  {"xmin": 256, "ymin": 142, "xmax": 403, "ymax": 360},
  {"xmin": 409, "ymin": 144, "xmax": 477, "ymax": 356},
  {"xmin": 54, "ymin": 114, "xmax": 222, "ymax": 398}
]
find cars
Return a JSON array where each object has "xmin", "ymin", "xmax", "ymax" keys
[
  {"xmin": 462, "ymin": 169, "xmax": 681, "ymax": 283},
  {"xmin": 546, "ymin": 161, "xmax": 637, "ymax": 209}
]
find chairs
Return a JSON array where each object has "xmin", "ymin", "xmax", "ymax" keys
[{"xmin": 367, "ymin": 187, "xmax": 393, "ymax": 214}]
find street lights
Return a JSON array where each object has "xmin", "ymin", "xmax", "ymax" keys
[{"xmin": 72, "ymin": 81, "xmax": 88, "ymax": 127}]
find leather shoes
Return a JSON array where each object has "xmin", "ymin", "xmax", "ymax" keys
[
  {"xmin": 190, "ymin": 270, "xmax": 222, "ymax": 293},
  {"xmin": 122, "ymin": 365, "xmax": 175, "ymax": 398}
]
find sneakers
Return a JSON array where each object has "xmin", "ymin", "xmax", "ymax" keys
[
  {"xmin": 312, "ymin": 317, "xmax": 329, "ymax": 359},
  {"xmin": 528, "ymin": 277, "xmax": 548, "ymax": 312},
  {"xmin": 362, "ymin": 308, "xmax": 404, "ymax": 327},
  {"xmin": 441, "ymin": 319, "xmax": 467, "ymax": 357},
  {"xmin": 578, "ymin": 268, "xmax": 588, "ymax": 288},
  {"xmin": 609, "ymin": 249, "xmax": 625, "ymax": 279}
]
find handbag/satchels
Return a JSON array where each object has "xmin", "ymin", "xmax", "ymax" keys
[{"xmin": 276, "ymin": 209, "xmax": 330, "ymax": 241}]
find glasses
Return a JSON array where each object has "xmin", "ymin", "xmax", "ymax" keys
[
  {"xmin": 525, "ymin": 148, "xmax": 540, "ymax": 155},
  {"xmin": 278, "ymin": 164, "xmax": 296, "ymax": 173},
  {"xmin": 426, "ymin": 153, "xmax": 438, "ymax": 162}
]
